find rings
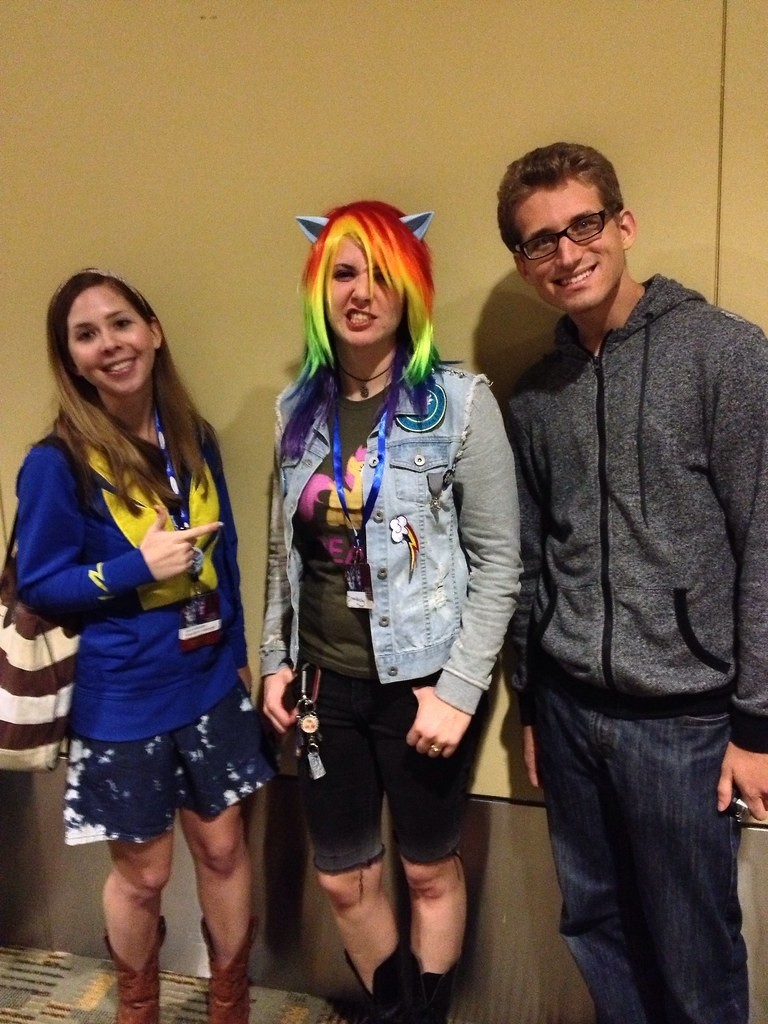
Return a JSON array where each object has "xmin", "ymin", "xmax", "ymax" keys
[{"xmin": 430, "ymin": 745, "xmax": 441, "ymax": 753}]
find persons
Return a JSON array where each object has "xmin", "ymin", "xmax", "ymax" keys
[
  {"xmin": 498, "ymin": 141, "xmax": 768, "ymax": 1024},
  {"xmin": 260, "ymin": 200, "xmax": 523, "ymax": 1024},
  {"xmin": 15, "ymin": 272, "xmax": 273, "ymax": 1024}
]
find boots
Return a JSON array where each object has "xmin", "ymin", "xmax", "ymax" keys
[
  {"xmin": 200, "ymin": 915, "xmax": 259, "ymax": 1024},
  {"xmin": 344, "ymin": 938, "xmax": 462, "ymax": 1024},
  {"xmin": 104, "ymin": 915, "xmax": 166, "ymax": 1024}
]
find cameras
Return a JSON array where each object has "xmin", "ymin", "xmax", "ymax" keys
[{"xmin": 729, "ymin": 792, "xmax": 751, "ymax": 824}]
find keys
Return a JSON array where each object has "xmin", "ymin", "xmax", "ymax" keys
[{"xmin": 294, "ymin": 699, "xmax": 323, "ymax": 761}]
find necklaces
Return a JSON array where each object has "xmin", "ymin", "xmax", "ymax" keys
[{"xmin": 336, "ymin": 359, "xmax": 394, "ymax": 398}]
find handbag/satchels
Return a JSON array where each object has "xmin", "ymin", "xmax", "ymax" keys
[{"xmin": 0, "ymin": 434, "xmax": 87, "ymax": 772}]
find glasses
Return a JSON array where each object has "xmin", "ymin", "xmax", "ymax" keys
[{"xmin": 514, "ymin": 202, "xmax": 619, "ymax": 260}]
